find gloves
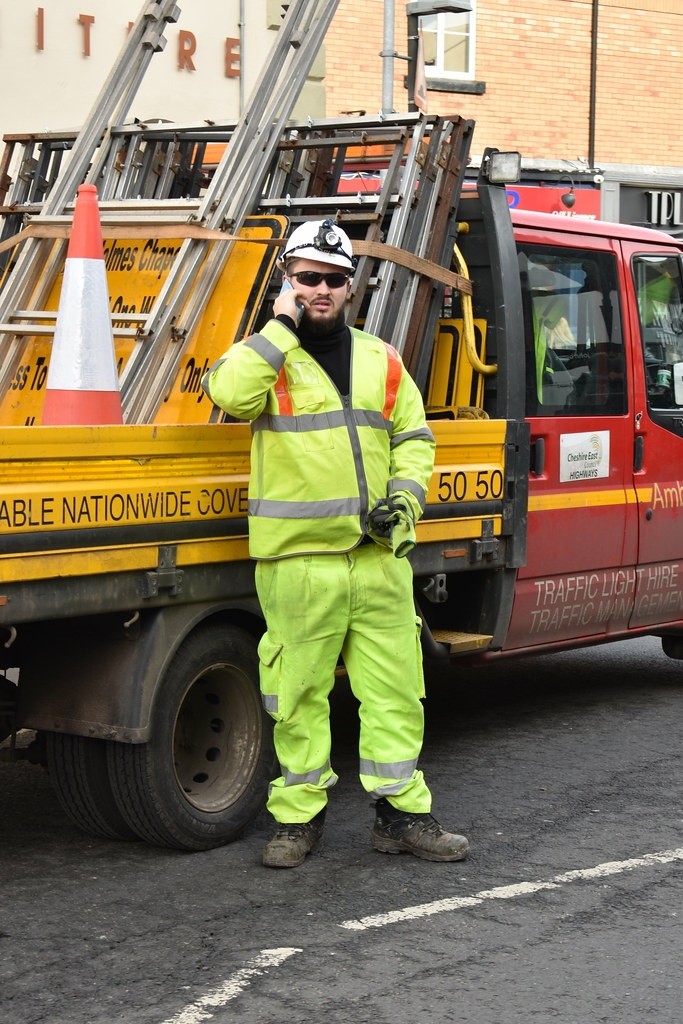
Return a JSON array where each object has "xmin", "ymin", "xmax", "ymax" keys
[{"xmin": 369, "ymin": 495, "xmax": 417, "ymax": 558}]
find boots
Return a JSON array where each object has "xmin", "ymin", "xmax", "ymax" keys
[
  {"xmin": 373, "ymin": 797, "xmax": 470, "ymax": 862},
  {"xmin": 262, "ymin": 804, "xmax": 326, "ymax": 867}
]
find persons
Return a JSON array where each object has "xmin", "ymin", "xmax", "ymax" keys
[{"xmin": 200, "ymin": 217, "xmax": 474, "ymax": 866}]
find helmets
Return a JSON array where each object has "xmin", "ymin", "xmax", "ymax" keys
[{"xmin": 275, "ymin": 219, "xmax": 355, "ymax": 273}]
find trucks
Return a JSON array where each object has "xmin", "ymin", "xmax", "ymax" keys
[{"xmin": 0, "ymin": 170, "xmax": 683, "ymax": 853}]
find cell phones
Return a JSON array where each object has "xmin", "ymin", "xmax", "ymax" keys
[{"xmin": 279, "ymin": 278, "xmax": 304, "ymax": 328}]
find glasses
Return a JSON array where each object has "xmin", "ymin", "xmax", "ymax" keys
[{"xmin": 288, "ymin": 271, "xmax": 349, "ymax": 289}]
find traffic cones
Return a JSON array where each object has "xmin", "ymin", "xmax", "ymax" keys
[{"xmin": 40, "ymin": 181, "xmax": 124, "ymax": 427}]
